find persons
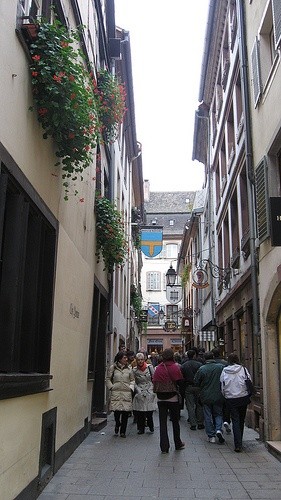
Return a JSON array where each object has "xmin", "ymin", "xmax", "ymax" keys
[
  {"xmin": 106, "ymin": 345, "xmax": 162, "ymax": 438},
  {"xmin": 152, "ymin": 348, "xmax": 185, "ymax": 454},
  {"xmin": 220, "ymin": 353, "xmax": 252, "ymax": 451},
  {"xmin": 170, "ymin": 347, "xmax": 232, "ymax": 443}
]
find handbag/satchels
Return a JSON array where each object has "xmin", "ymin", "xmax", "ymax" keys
[
  {"xmin": 176, "ymin": 385, "xmax": 182, "ymax": 405},
  {"xmin": 244, "ymin": 367, "xmax": 257, "ymax": 397}
]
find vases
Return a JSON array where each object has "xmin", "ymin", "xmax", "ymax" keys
[{"xmin": 23, "ymin": 22, "xmax": 40, "ymax": 38}]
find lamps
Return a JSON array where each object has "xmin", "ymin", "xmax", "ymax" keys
[
  {"xmin": 158, "ymin": 306, "xmax": 166, "ymax": 321},
  {"xmin": 165, "ymin": 264, "xmax": 182, "ymax": 288}
]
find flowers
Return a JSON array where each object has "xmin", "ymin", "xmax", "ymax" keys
[
  {"xmin": 130, "ymin": 284, "xmax": 142, "ymax": 314},
  {"xmin": 11, "ymin": 3, "xmax": 143, "ymax": 275}
]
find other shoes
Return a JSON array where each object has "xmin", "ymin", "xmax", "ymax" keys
[
  {"xmin": 120, "ymin": 433, "xmax": 124, "ymax": 437},
  {"xmin": 149, "ymin": 426, "xmax": 154, "ymax": 432},
  {"xmin": 215, "ymin": 430, "xmax": 225, "ymax": 443},
  {"xmin": 137, "ymin": 430, "xmax": 145, "ymax": 434},
  {"xmin": 161, "ymin": 448, "xmax": 168, "ymax": 454},
  {"xmin": 234, "ymin": 447, "xmax": 240, "ymax": 451},
  {"xmin": 115, "ymin": 427, "xmax": 119, "ymax": 433},
  {"xmin": 190, "ymin": 426, "xmax": 196, "ymax": 430},
  {"xmin": 207, "ymin": 437, "xmax": 215, "ymax": 443},
  {"xmin": 175, "ymin": 442, "xmax": 184, "ymax": 450},
  {"xmin": 224, "ymin": 422, "xmax": 231, "ymax": 433},
  {"xmin": 197, "ymin": 424, "xmax": 205, "ymax": 429}
]
